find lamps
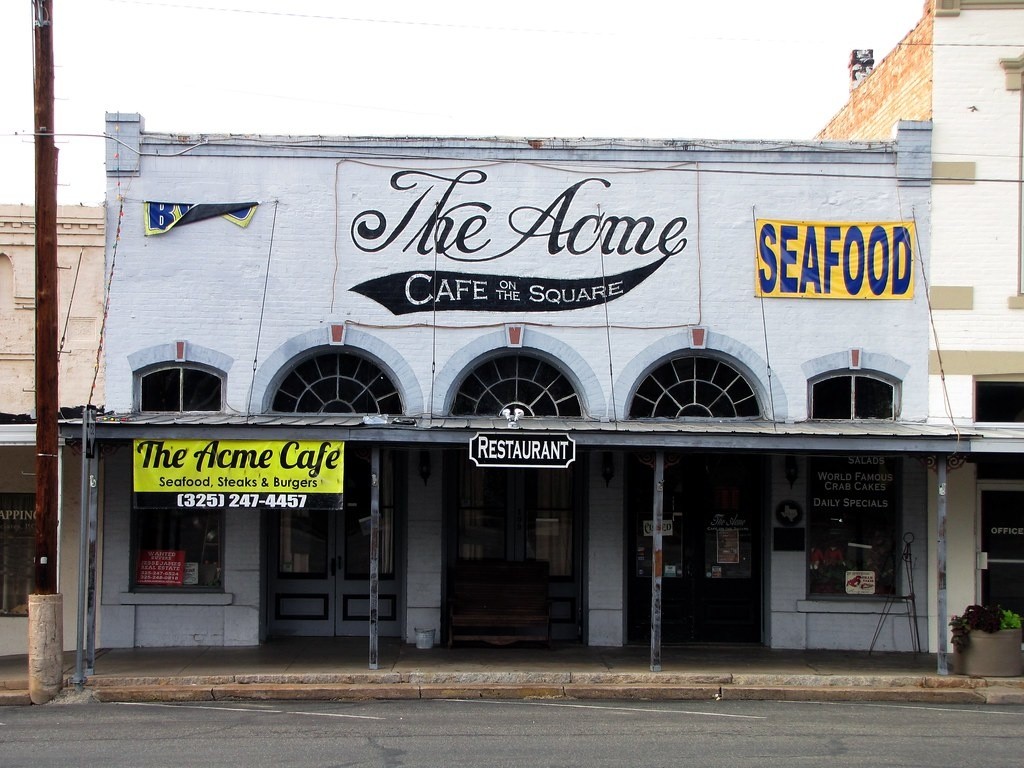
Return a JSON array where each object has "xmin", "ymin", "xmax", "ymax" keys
[
  {"xmin": 784, "ymin": 456, "xmax": 798, "ymax": 489},
  {"xmin": 420, "ymin": 450, "xmax": 431, "ymax": 486},
  {"xmin": 602, "ymin": 452, "xmax": 614, "ymax": 488}
]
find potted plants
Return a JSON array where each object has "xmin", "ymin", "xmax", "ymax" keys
[{"xmin": 948, "ymin": 604, "xmax": 1022, "ymax": 676}]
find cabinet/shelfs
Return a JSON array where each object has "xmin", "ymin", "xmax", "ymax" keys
[{"xmin": 201, "ymin": 515, "xmax": 221, "ymax": 585}]
